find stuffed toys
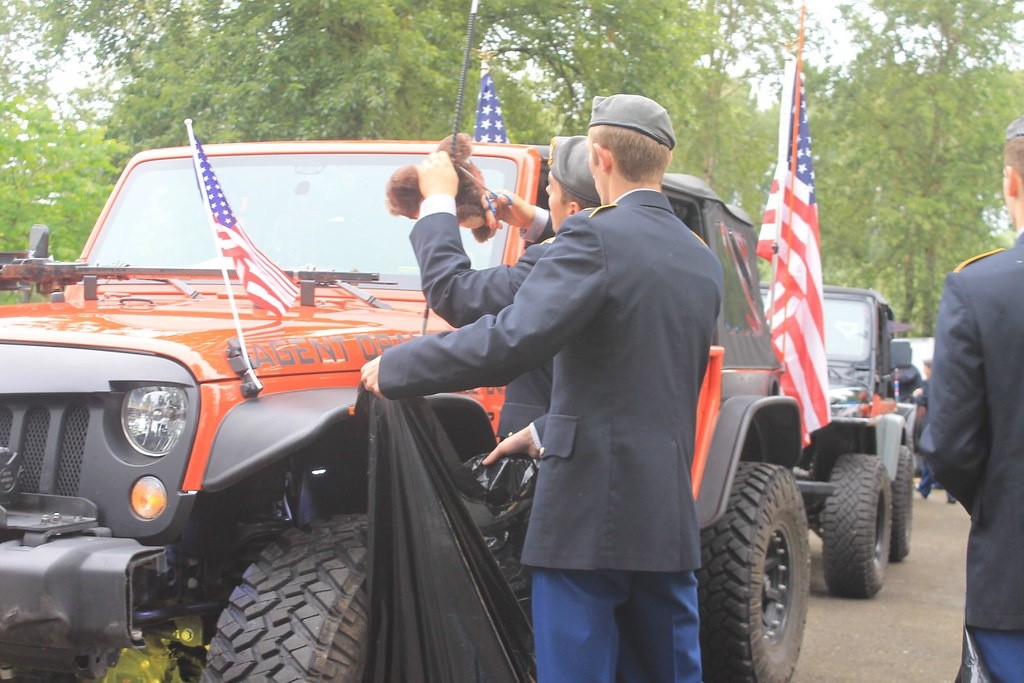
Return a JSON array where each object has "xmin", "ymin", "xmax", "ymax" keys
[{"xmin": 387, "ymin": 131, "xmax": 504, "ymax": 243}]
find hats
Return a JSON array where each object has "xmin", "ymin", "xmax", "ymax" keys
[
  {"xmin": 588, "ymin": 94, "xmax": 676, "ymax": 150},
  {"xmin": 1006, "ymin": 118, "xmax": 1024, "ymax": 140},
  {"xmin": 548, "ymin": 135, "xmax": 601, "ymax": 202}
]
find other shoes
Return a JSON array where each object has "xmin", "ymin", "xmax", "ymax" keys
[{"xmin": 914, "ymin": 483, "xmax": 927, "ymax": 498}]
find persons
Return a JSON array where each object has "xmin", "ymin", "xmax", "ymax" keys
[
  {"xmin": 411, "ymin": 136, "xmax": 605, "ymax": 450},
  {"xmin": 358, "ymin": 94, "xmax": 721, "ymax": 682},
  {"xmin": 473, "ymin": 65, "xmax": 513, "ymax": 146},
  {"xmin": 911, "ymin": 116, "xmax": 1024, "ymax": 683}
]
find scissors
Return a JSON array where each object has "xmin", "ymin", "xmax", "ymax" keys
[{"xmin": 458, "ymin": 167, "xmax": 512, "ymax": 219}]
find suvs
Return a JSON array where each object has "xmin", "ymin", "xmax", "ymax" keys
[
  {"xmin": 0, "ymin": 141, "xmax": 810, "ymax": 682},
  {"xmin": 761, "ymin": 285, "xmax": 941, "ymax": 600}
]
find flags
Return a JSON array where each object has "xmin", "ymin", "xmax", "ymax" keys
[
  {"xmin": 192, "ymin": 133, "xmax": 301, "ymax": 317},
  {"xmin": 754, "ymin": 53, "xmax": 833, "ymax": 449}
]
